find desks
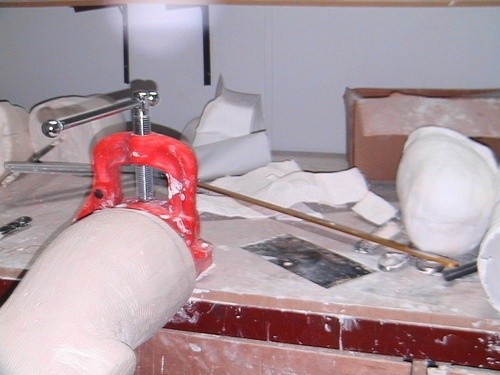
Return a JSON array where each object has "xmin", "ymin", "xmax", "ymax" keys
[{"xmin": 1, "ymin": 146, "xmax": 500, "ymax": 374}]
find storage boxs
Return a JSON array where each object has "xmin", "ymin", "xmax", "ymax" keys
[{"xmin": 343, "ymin": 87, "xmax": 500, "ymax": 183}]
